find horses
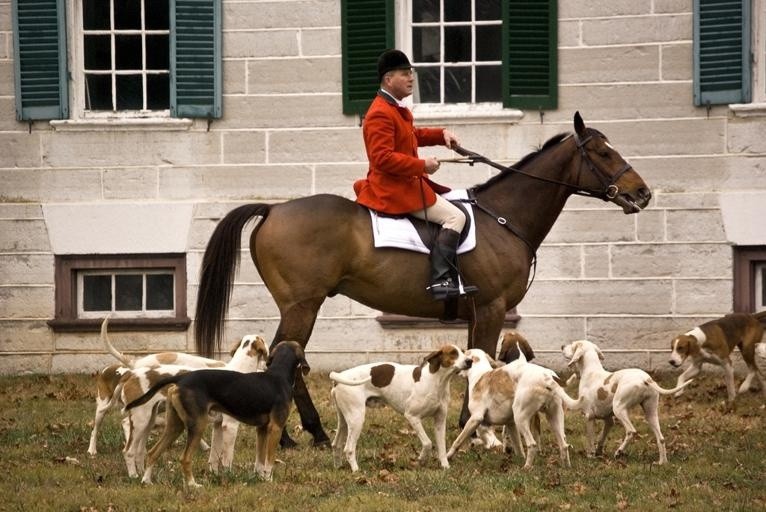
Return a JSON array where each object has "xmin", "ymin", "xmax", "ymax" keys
[{"xmin": 192, "ymin": 110, "xmax": 653, "ymax": 449}]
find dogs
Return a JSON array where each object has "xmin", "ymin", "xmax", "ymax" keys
[
  {"xmin": 498, "ymin": 330, "xmax": 551, "ymax": 454},
  {"xmin": 665, "ymin": 307, "xmax": 766, "ymax": 403},
  {"xmin": 325, "ymin": 343, "xmax": 474, "ymax": 475},
  {"xmin": 86, "ymin": 311, "xmax": 267, "ymax": 479},
  {"xmin": 561, "ymin": 337, "xmax": 694, "ymax": 466},
  {"xmin": 119, "ymin": 341, "xmax": 310, "ymax": 488},
  {"xmin": 445, "ymin": 346, "xmax": 571, "ymax": 470}
]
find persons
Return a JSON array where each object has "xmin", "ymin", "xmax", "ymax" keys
[{"xmin": 351, "ymin": 48, "xmax": 480, "ymax": 305}]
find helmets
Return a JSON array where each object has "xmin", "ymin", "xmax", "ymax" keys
[{"xmin": 378, "ymin": 50, "xmax": 418, "ymax": 84}]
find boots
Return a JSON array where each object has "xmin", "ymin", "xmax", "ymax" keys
[{"xmin": 429, "ymin": 247, "xmax": 479, "ymax": 301}]
